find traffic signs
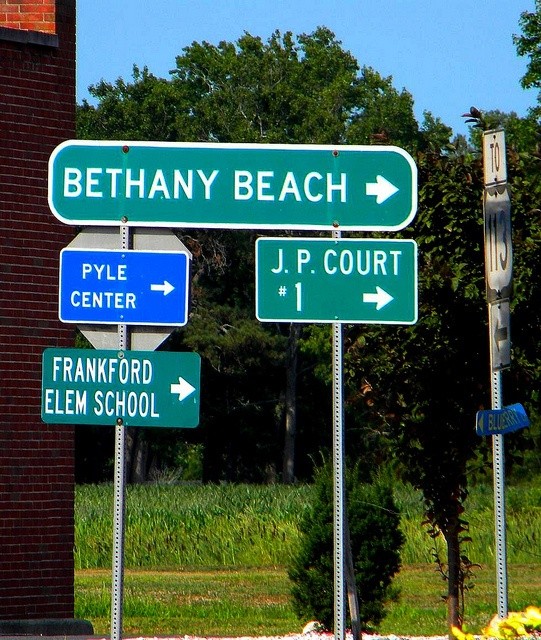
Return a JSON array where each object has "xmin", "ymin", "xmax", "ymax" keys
[
  {"xmin": 48, "ymin": 138, "xmax": 417, "ymax": 232},
  {"xmin": 67, "ymin": 228, "xmax": 192, "ymax": 351},
  {"xmin": 41, "ymin": 348, "xmax": 201, "ymax": 428},
  {"xmin": 475, "ymin": 403, "xmax": 530, "ymax": 436},
  {"xmin": 253, "ymin": 237, "xmax": 417, "ymax": 325},
  {"xmin": 59, "ymin": 248, "xmax": 189, "ymax": 327},
  {"xmin": 485, "ymin": 190, "xmax": 513, "ymax": 292}
]
